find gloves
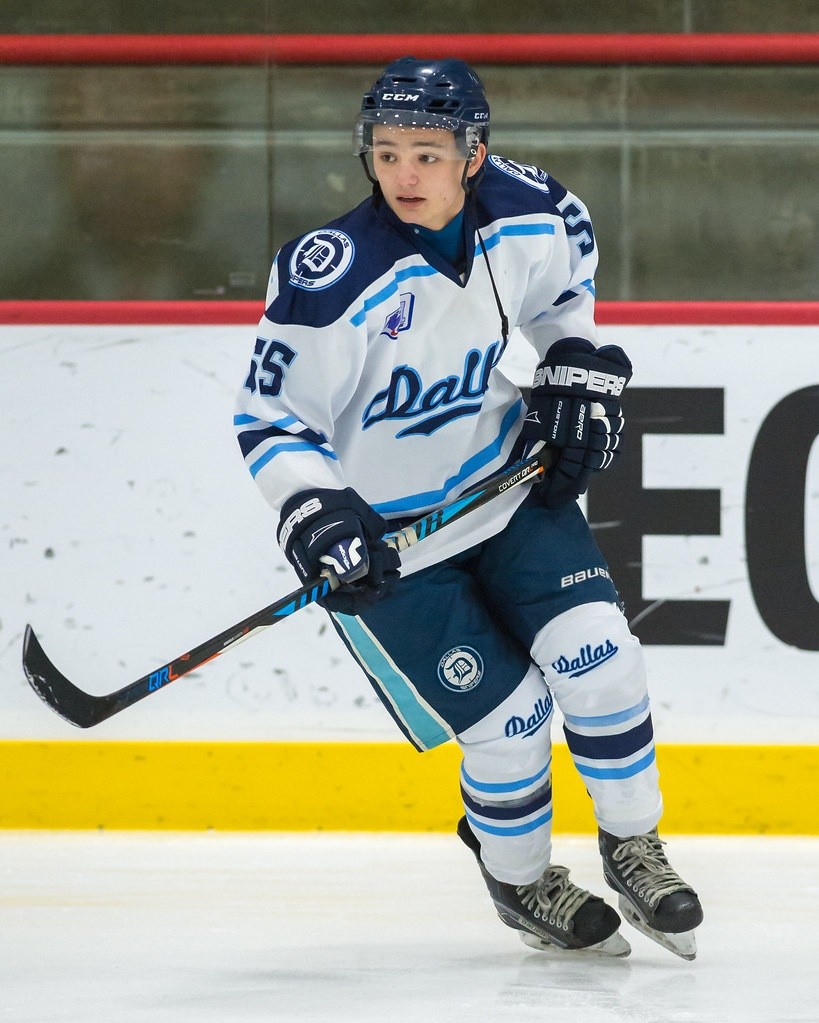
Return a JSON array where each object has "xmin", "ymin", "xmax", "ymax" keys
[
  {"xmin": 524, "ymin": 337, "xmax": 633, "ymax": 511},
  {"xmin": 276, "ymin": 487, "xmax": 402, "ymax": 617}
]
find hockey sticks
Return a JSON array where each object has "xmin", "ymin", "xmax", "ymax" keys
[{"xmin": 20, "ymin": 447, "xmax": 547, "ymax": 731}]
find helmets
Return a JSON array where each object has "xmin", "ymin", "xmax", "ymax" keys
[{"xmin": 362, "ymin": 57, "xmax": 490, "ymax": 146}]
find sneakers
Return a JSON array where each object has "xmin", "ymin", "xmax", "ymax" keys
[
  {"xmin": 457, "ymin": 815, "xmax": 631, "ymax": 957},
  {"xmin": 598, "ymin": 826, "xmax": 703, "ymax": 961}
]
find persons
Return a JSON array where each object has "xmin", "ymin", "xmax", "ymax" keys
[{"xmin": 234, "ymin": 55, "xmax": 703, "ymax": 950}]
